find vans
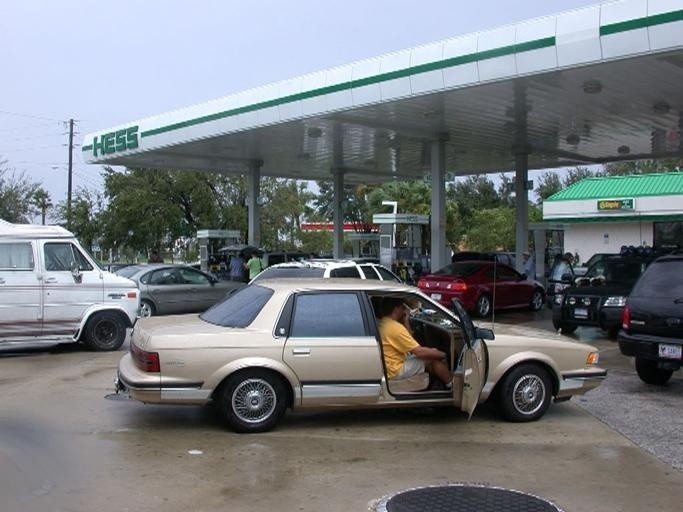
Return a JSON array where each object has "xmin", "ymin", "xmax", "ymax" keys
[{"xmin": 0, "ymin": 223, "xmax": 140, "ymax": 353}]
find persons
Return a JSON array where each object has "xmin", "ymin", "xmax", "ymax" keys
[
  {"xmin": 543, "ymin": 247, "xmax": 575, "ymax": 286},
  {"xmin": 148, "ymin": 250, "xmax": 164, "ymax": 263},
  {"xmin": 221, "ymin": 249, "xmax": 268, "ymax": 282},
  {"xmin": 377, "ymin": 297, "xmax": 457, "ymax": 389}
]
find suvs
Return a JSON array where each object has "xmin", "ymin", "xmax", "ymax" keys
[{"xmin": 545, "ymin": 244, "xmax": 682, "ymax": 386}]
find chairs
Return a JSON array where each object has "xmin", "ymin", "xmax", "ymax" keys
[{"xmin": 388, "ymin": 371, "xmax": 430, "ymax": 392}]
